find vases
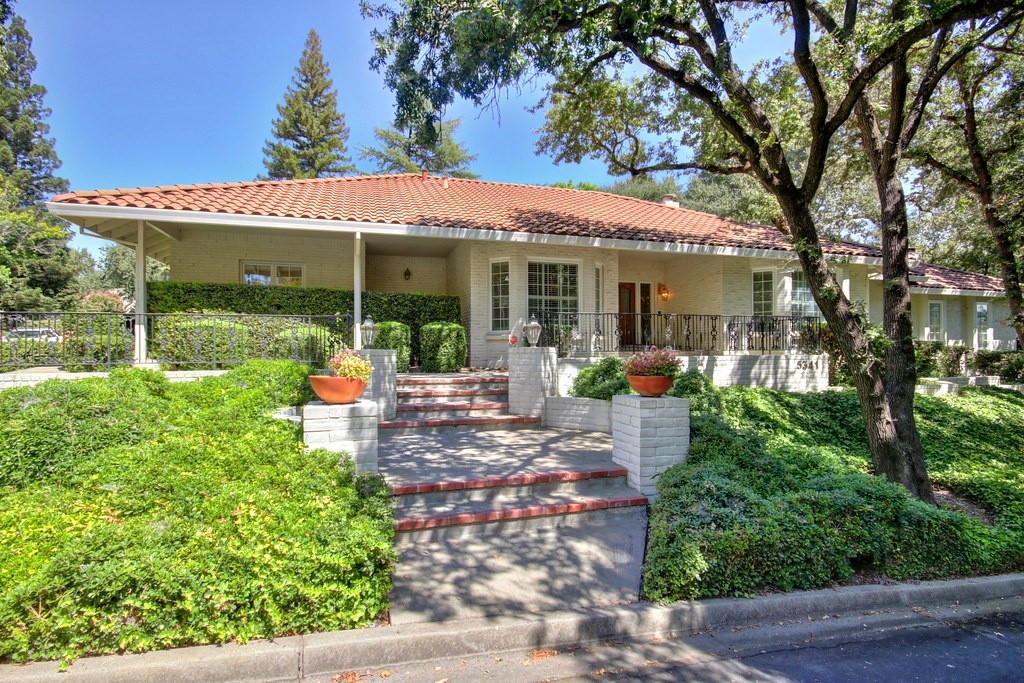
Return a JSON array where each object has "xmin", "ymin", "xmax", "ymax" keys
[
  {"xmin": 309, "ymin": 375, "xmax": 368, "ymax": 403},
  {"xmin": 626, "ymin": 373, "xmax": 675, "ymax": 397}
]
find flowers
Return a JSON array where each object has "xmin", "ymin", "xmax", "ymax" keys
[
  {"xmin": 327, "ymin": 348, "xmax": 375, "ymax": 386},
  {"xmin": 620, "ymin": 343, "xmax": 685, "ymax": 377}
]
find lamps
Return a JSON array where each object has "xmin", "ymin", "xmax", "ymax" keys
[
  {"xmin": 522, "ymin": 312, "xmax": 543, "ymax": 348},
  {"xmin": 404, "ymin": 267, "xmax": 410, "ymax": 280},
  {"xmin": 657, "ymin": 281, "xmax": 669, "ymax": 303},
  {"xmin": 359, "ymin": 313, "xmax": 380, "ymax": 351}
]
